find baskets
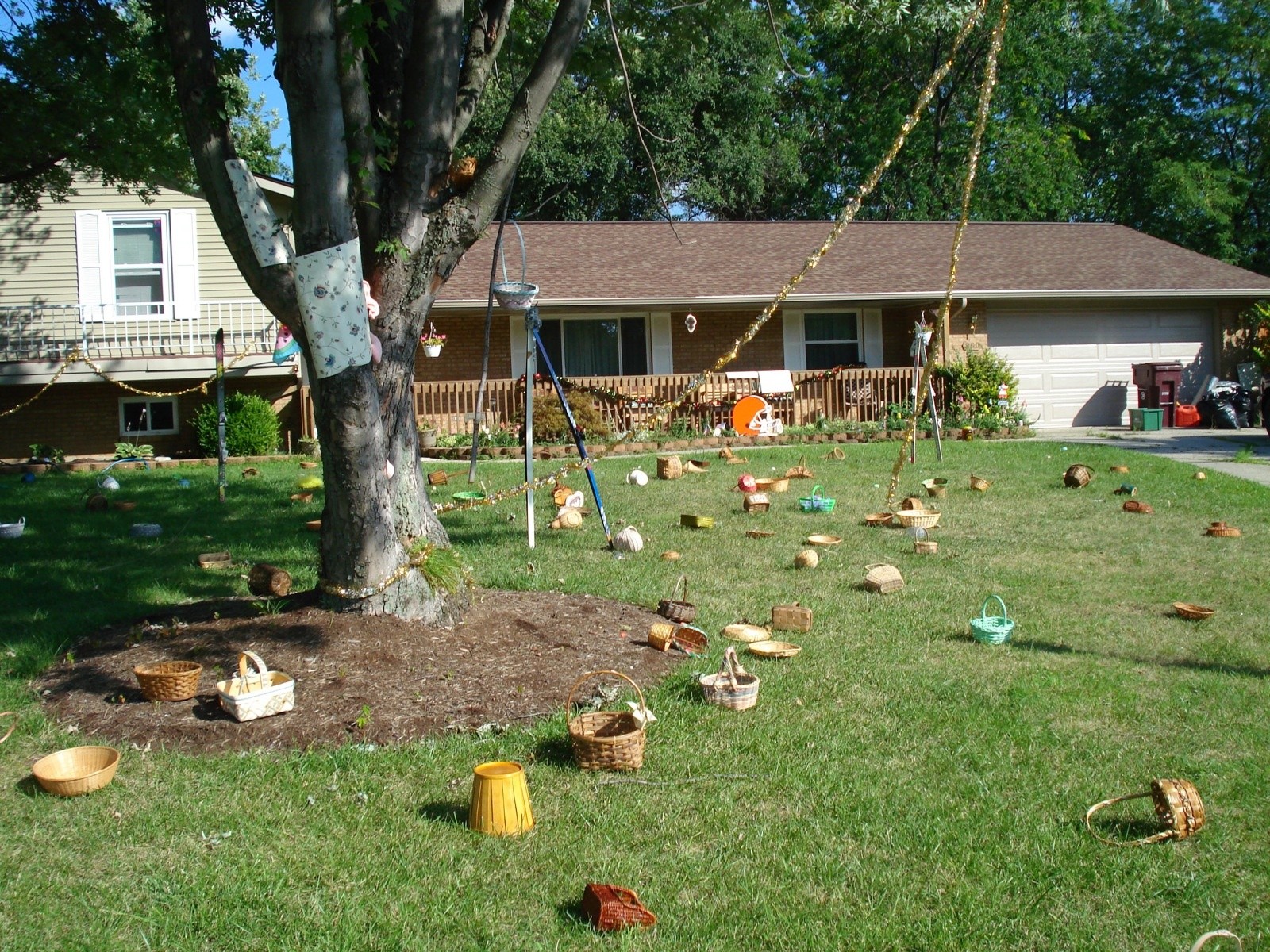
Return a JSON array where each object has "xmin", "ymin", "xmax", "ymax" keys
[
  {"xmin": 200, "ymin": 552, "xmax": 233, "ymax": 569},
  {"xmin": 241, "ymin": 467, "xmax": 259, "ymax": 478},
  {"xmin": 685, "ymin": 445, "xmax": 1245, "ymax": 568},
  {"xmin": 772, "ymin": 602, "xmax": 812, "ymax": 633},
  {"xmin": 427, "ymin": 469, "xmax": 468, "ymax": 485},
  {"xmin": 657, "ymin": 456, "xmax": 689, "ymax": 480},
  {"xmin": 1085, "ymin": 778, "xmax": 1206, "ymax": 844},
  {"xmin": 215, "ymin": 651, "xmax": 295, "ymax": 722},
  {"xmin": 291, "ymin": 493, "xmax": 312, "ymax": 503},
  {"xmin": 243, "ymin": 564, "xmax": 292, "ymax": 597},
  {"xmin": 133, "ymin": 661, "xmax": 203, "ymax": 702},
  {"xmin": 453, "ymin": 490, "xmax": 486, "ymax": 503},
  {"xmin": 675, "ymin": 626, "xmax": 709, "ymax": 654},
  {"xmin": 658, "ymin": 574, "xmax": 695, "ymax": 623},
  {"xmin": 306, "ymin": 520, "xmax": 321, "ymax": 529},
  {"xmin": 82, "ymin": 471, "xmax": 163, "ymax": 537},
  {"xmin": 613, "ymin": 526, "xmax": 643, "ymax": 552},
  {"xmin": 32, "ymin": 745, "xmax": 120, "ymax": 796},
  {"xmin": 550, "ymin": 511, "xmax": 581, "ymax": 529},
  {"xmin": 864, "ymin": 563, "xmax": 905, "ymax": 594},
  {"xmin": 969, "ymin": 595, "xmax": 1015, "ymax": 645},
  {"xmin": 581, "ymin": 884, "xmax": 655, "ymax": 929},
  {"xmin": 1172, "ymin": 599, "xmax": 1216, "ymax": 620},
  {"xmin": 700, "ymin": 647, "xmax": 759, "ymax": 710},
  {"xmin": 0, "ymin": 517, "xmax": 24, "ymax": 538},
  {"xmin": 647, "ymin": 621, "xmax": 674, "ymax": 651},
  {"xmin": 566, "ymin": 670, "xmax": 647, "ymax": 770},
  {"xmin": 300, "ymin": 462, "xmax": 317, "ymax": 469}
]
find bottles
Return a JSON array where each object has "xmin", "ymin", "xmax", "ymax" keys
[{"xmin": 1175, "ymin": 402, "xmax": 1200, "ymax": 427}]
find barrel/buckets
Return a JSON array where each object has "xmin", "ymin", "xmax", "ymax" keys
[
  {"xmin": 468, "ymin": 760, "xmax": 534, "ymax": 838},
  {"xmin": 630, "ymin": 470, "xmax": 648, "ymax": 486},
  {"xmin": 22, "ymin": 472, "xmax": 35, "ymax": 483}
]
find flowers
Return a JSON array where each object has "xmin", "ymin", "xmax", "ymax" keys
[{"xmin": 420, "ymin": 331, "xmax": 447, "ymax": 348}]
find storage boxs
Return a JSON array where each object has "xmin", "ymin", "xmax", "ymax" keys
[{"xmin": 1127, "ymin": 406, "xmax": 1165, "ymax": 433}]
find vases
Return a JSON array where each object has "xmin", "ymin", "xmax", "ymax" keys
[{"xmin": 422, "ymin": 346, "xmax": 443, "ymax": 358}]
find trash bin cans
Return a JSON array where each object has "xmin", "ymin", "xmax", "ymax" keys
[{"xmin": 1132, "ymin": 361, "xmax": 1183, "ymax": 428}]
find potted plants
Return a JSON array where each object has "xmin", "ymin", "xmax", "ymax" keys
[
  {"xmin": 533, "ymin": 441, "xmax": 544, "ymax": 460},
  {"xmin": 503, "ymin": 431, "xmax": 525, "ymax": 458},
  {"xmin": 484, "ymin": 431, "xmax": 505, "ymax": 459},
  {"xmin": 470, "ymin": 432, "xmax": 485, "ymax": 456},
  {"xmin": 457, "ymin": 434, "xmax": 469, "ymax": 459},
  {"xmin": 297, "ymin": 433, "xmax": 319, "ymax": 455},
  {"xmin": 439, "ymin": 434, "xmax": 454, "ymax": 458},
  {"xmin": 418, "ymin": 417, "xmax": 438, "ymax": 449},
  {"xmin": 589, "ymin": 407, "xmax": 1034, "ymax": 456},
  {"xmin": 539, "ymin": 437, "xmax": 567, "ymax": 458},
  {"xmin": 114, "ymin": 438, "xmax": 156, "ymax": 472},
  {"xmin": 23, "ymin": 438, "xmax": 65, "ymax": 474}
]
